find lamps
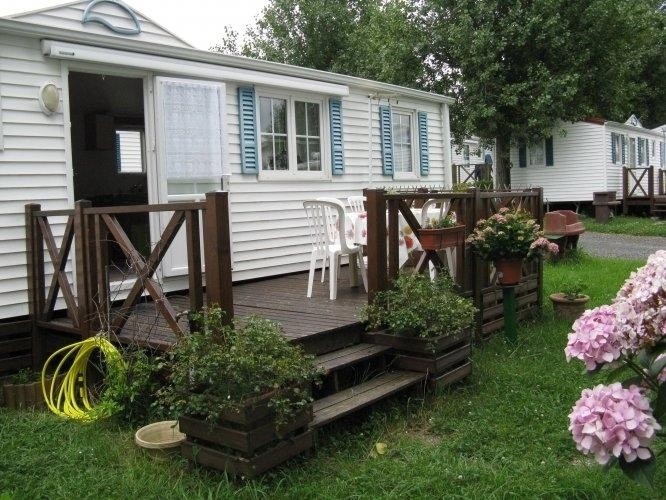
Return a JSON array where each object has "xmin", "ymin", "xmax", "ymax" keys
[{"xmin": 38, "ymin": 82, "xmax": 59, "ymax": 117}]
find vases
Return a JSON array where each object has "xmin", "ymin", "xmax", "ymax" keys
[{"xmin": 493, "ymin": 259, "xmax": 524, "ymax": 284}]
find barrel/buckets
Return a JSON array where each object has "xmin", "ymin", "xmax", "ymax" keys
[{"xmin": 593, "ymin": 190, "xmax": 616, "ymax": 223}]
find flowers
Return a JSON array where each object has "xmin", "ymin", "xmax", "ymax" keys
[
  {"xmin": 463, "ymin": 206, "xmax": 559, "ymax": 260},
  {"xmin": 563, "ymin": 248, "xmax": 666, "ymax": 492}
]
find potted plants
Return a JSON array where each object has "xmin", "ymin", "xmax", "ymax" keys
[
  {"xmin": 416, "ymin": 211, "xmax": 466, "ymax": 251},
  {"xmin": 361, "ymin": 263, "xmax": 481, "ymax": 396},
  {"xmin": 0, "ymin": 366, "xmax": 69, "ymax": 410},
  {"xmin": 548, "ymin": 282, "xmax": 591, "ymax": 322},
  {"xmin": 150, "ymin": 301, "xmax": 325, "ymax": 486}
]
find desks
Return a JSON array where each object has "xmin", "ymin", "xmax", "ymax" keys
[{"xmin": 334, "ymin": 208, "xmax": 459, "ymax": 288}]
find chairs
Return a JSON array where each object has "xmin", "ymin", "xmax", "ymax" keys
[
  {"xmin": 413, "ymin": 198, "xmax": 460, "ymax": 283},
  {"xmin": 347, "ymin": 195, "xmax": 370, "ymax": 213},
  {"xmin": 302, "ymin": 197, "xmax": 368, "ymax": 301}
]
